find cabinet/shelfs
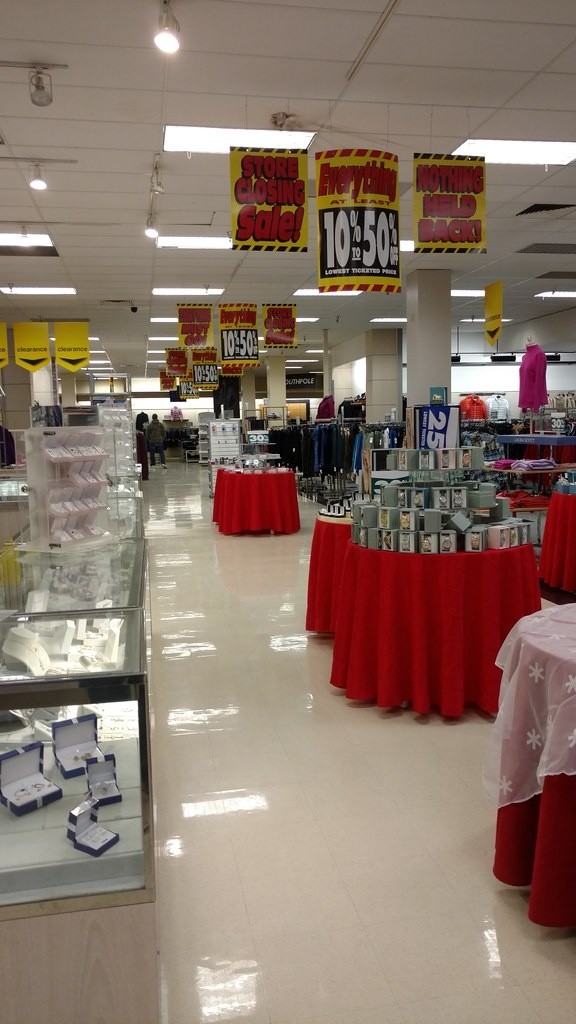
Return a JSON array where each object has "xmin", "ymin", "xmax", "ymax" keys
[{"xmin": 12, "ymin": 426, "xmax": 116, "ymax": 554}]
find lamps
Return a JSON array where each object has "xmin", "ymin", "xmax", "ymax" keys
[
  {"xmin": 26, "ymin": 66, "xmax": 55, "ymax": 107},
  {"xmin": 27, "ymin": 160, "xmax": 48, "ymax": 191},
  {"xmin": 154, "ymin": 1, "xmax": 181, "ymax": 53},
  {"xmin": 149, "ymin": 168, "xmax": 167, "ymax": 197},
  {"xmin": 144, "ymin": 210, "xmax": 158, "ymax": 239},
  {"xmin": 17, "ymin": 225, "xmax": 31, "ymax": 247}
]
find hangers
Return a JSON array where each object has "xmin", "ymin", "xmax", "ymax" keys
[{"xmin": 162, "ymin": 393, "xmax": 576, "ymax": 456}]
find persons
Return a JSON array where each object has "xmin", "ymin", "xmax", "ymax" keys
[
  {"xmin": 147, "ymin": 413, "xmax": 168, "ymax": 468},
  {"xmin": 317, "ymin": 394, "xmax": 335, "ymax": 424},
  {"xmin": 517, "ymin": 343, "xmax": 548, "ymax": 435}
]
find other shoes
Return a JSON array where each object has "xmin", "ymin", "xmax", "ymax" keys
[
  {"xmin": 162, "ymin": 464, "xmax": 167, "ymax": 469},
  {"xmin": 152, "ymin": 465, "xmax": 157, "ymax": 469}
]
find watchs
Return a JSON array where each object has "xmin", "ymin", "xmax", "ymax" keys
[{"xmin": 358, "ymin": 449, "xmax": 516, "ymax": 550}]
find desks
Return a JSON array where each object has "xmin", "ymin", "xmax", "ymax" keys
[
  {"xmin": 212, "ymin": 467, "xmax": 301, "ymax": 536},
  {"xmin": 94, "ymin": 403, "xmax": 134, "ymax": 478},
  {"xmin": 538, "ymin": 490, "xmax": 576, "ymax": 594},
  {"xmin": 328, "ymin": 534, "xmax": 543, "ymax": 717},
  {"xmin": 493, "ymin": 602, "xmax": 576, "ymax": 928},
  {"xmin": 304, "ymin": 509, "xmax": 357, "ymax": 635}
]
